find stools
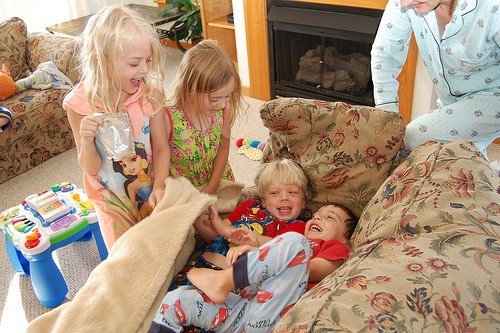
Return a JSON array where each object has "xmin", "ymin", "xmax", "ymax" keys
[{"xmin": 0, "ymin": 182, "xmax": 108, "ymax": 308}]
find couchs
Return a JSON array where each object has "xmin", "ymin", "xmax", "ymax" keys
[
  {"xmin": 0, "ymin": 15, "xmax": 80, "ymax": 183},
  {"xmin": 257, "ymin": 92, "xmax": 500, "ymax": 333}
]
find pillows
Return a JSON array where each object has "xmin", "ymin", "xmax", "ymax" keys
[{"xmin": 261, "ymin": 98, "xmax": 407, "ymax": 219}]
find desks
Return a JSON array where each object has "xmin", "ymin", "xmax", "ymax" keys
[{"xmin": 46, "ymin": 4, "xmax": 189, "ymax": 56}]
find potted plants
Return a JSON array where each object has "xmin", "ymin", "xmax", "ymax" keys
[{"xmin": 160, "ymin": 0, "xmax": 204, "ymax": 47}]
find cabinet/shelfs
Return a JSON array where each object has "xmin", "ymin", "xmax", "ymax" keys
[{"xmin": 200, "ymin": 0, "xmax": 419, "ymax": 127}]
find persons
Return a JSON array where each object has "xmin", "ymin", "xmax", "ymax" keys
[
  {"xmin": 165, "ymin": 41, "xmax": 240, "ymax": 195},
  {"xmin": 148, "ymin": 203, "xmax": 357, "ymax": 333},
  {"xmin": 169, "ymin": 160, "xmax": 307, "ymax": 290},
  {"xmin": 63, "ymin": 5, "xmax": 171, "ymax": 254},
  {"xmin": 371, "ymin": 0, "xmax": 499, "ymax": 152}
]
